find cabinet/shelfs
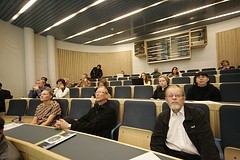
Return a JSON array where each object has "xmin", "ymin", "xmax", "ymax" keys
[{"xmin": 134, "ymin": 26, "xmax": 208, "ymax": 64}]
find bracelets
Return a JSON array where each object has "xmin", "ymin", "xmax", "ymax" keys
[{"xmin": 67, "ymin": 124, "xmax": 72, "ymax": 130}]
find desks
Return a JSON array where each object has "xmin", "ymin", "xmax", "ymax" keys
[{"xmin": 3, "ymin": 70, "xmax": 240, "ymax": 160}]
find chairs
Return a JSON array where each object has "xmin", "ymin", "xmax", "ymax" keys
[{"xmin": 6, "ymin": 66, "xmax": 240, "ymax": 160}]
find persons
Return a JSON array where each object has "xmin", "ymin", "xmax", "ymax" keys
[
  {"xmin": 53, "ymin": 79, "xmax": 70, "ymax": 98},
  {"xmin": 90, "ymin": 65, "xmax": 103, "ymax": 79},
  {"xmin": 31, "ymin": 88, "xmax": 61, "ymax": 127},
  {"xmin": 150, "ymin": 75, "xmax": 170, "ymax": 100},
  {"xmin": 150, "ymin": 84, "xmax": 220, "ymax": 160},
  {"xmin": 220, "ymin": 60, "xmax": 235, "ymax": 70},
  {"xmin": 141, "ymin": 73, "xmax": 151, "ymax": 85},
  {"xmin": 74, "ymin": 73, "xmax": 91, "ymax": 88},
  {"xmin": 28, "ymin": 80, "xmax": 50, "ymax": 98},
  {"xmin": 93, "ymin": 78, "xmax": 111, "ymax": 98},
  {"xmin": 41, "ymin": 77, "xmax": 51, "ymax": 88},
  {"xmin": 151, "ymin": 67, "xmax": 162, "ymax": 78},
  {"xmin": 0, "ymin": 82, "xmax": 13, "ymax": 112},
  {"xmin": 168, "ymin": 67, "xmax": 182, "ymax": 78},
  {"xmin": 54, "ymin": 86, "xmax": 117, "ymax": 139},
  {"xmin": 186, "ymin": 72, "xmax": 222, "ymax": 102},
  {"xmin": 0, "ymin": 116, "xmax": 24, "ymax": 160},
  {"xmin": 63, "ymin": 78, "xmax": 71, "ymax": 87}
]
